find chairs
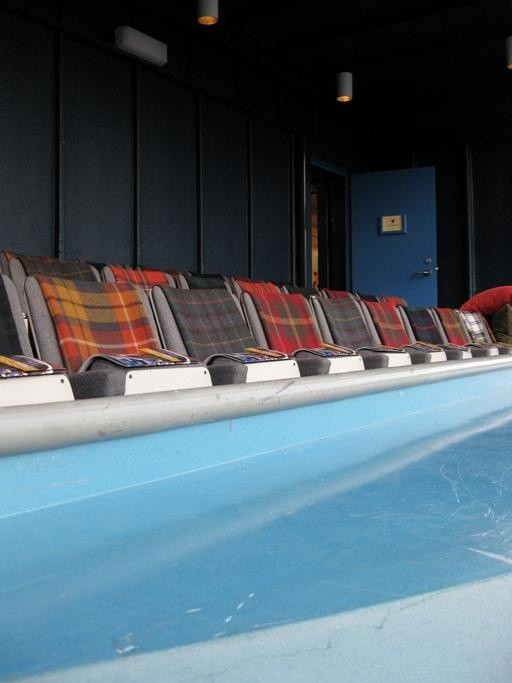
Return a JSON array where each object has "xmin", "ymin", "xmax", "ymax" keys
[{"xmin": 0, "ymin": 249, "xmax": 512, "ymax": 406}]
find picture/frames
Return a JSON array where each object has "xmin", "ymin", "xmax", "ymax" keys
[{"xmin": 378, "ymin": 214, "xmax": 407, "ymax": 236}]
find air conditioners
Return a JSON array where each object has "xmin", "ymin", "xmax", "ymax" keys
[{"xmin": 116, "ymin": 25, "xmax": 167, "ymax": 67}]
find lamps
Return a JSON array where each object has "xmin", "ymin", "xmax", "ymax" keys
[
  {"xmin": 336, "ymin": 71, "xmax": 352, "ymax": 102},
  {"xmin": 198, "ymin": 0, "xmax": 219, "ymax": 25}
]
